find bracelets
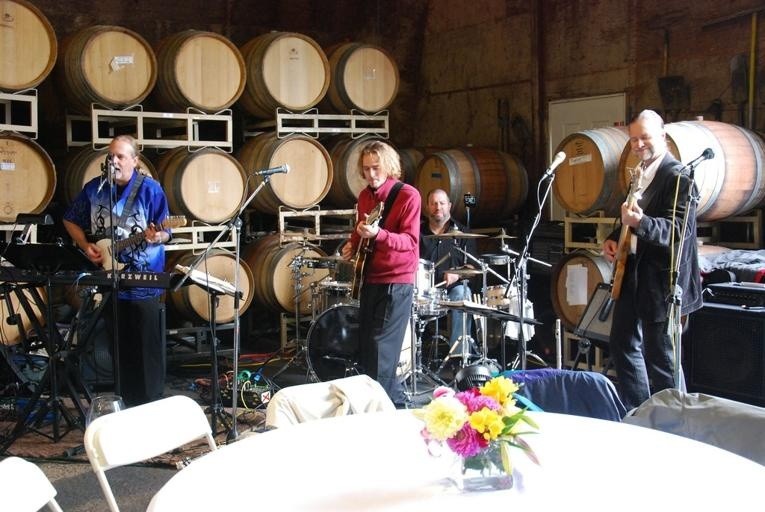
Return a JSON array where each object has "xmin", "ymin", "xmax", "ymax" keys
[{"xmin": 156, "ymin": 232, "xmax": 162, "ymax": 245}]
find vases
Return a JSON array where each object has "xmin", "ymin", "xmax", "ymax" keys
[{"xmin": 458, "ymin": 440, "xmax": 515, "ymax": 493}]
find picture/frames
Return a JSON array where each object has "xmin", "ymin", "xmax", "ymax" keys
[{"xmin": 547, "ymin": 91, "xmax": 630, "ymax": 222}]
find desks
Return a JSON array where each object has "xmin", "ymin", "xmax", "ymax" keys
[{"xmin": 142, "ymin": 404, "xmax": 764, "ymax": 512}]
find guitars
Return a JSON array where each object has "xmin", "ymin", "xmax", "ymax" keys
[
  {"xmin": 611, "ymin": 167, "xmax": 644, "ymax": 302},
  {"xmin": 350, "ymin": 203, "xmax": 382, "ymax": 296},
  {"xmin": 68, "ymin": 214, "xmax": 185, "ymax": 310}
]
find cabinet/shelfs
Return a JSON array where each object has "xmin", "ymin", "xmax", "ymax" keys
[
  {"xmin": 0, "ymin": 84, "xmax": 42, "ymax": 248},
  {"xmin": 554, "ymin": 210, "xmax": 765, "ymax": 395},
  {"xmin": 66, "ymin": 102, "xmax": 240, "ymax": 367},
  {"xmin": 243, "ymin": 104, "xmax": 393, "ymax": 361}
]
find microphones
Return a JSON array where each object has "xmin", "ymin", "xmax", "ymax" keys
[
  {"xmin": 96, "ymin": 164, "xmax": 115, "ymax": 195},
  {"xmin": 452, "ymin": 222, "xmax": 458, "ymax": 245},
  {"xmin": 255, "ymin": 162, "xmax": 290, "ymax": 176},
  {"xmin": 679, "ymin": 147, "xmax": 715, "ymax": 174},
  {"xmin": 542, "ymin": 151, "xmax": 567, "ymax": 180},
  {"xmin": 303, "ymin": 227, "xmax": 309, "ymax": 246}
]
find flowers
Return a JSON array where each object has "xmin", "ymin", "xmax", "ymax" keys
[{"xmin": 410, "ymin": 374, "xmax": 543, "ymax": 474}]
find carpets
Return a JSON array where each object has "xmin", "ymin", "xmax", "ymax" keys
[{"xmin": 0, "ymin": 387, "xmax": 273, "ymax": 473}]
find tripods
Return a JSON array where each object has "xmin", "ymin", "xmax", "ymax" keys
[
  {"xmin": 434, "ymin": 279, "xmax": 489, "ymax": 378},
  {"xmin": 468, "ymin": 261, "xmax": 503, "ymax": 371},
  {"xmin": 204, "ymin": 294, "xmax": 248, "ymax": 440},
  {"xmin": 396, "ymin": 303, "xmax": 441, "ymax": 397},
  {"xmin": 270, "ymin": 281, "xmax": 321, "ymax": 384},
  {"xmin": 15, "ymin": 260, "xmax": 87, "ymax": 444},
  {"xmin": 255, "ymin": 255, "xmax": 309, "ymax": 372},
  {"xmin": 511, "ymin": 251, "xmax": 553, "ymax": 371}
]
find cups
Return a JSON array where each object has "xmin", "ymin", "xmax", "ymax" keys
[{"xmin": 84, "ymin": 393, "xmax": 126, "ymax": 437}]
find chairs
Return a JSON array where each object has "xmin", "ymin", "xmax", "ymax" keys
[
  {"xmin": 261, "ymin": 373, "xmax": 400, "ymax": 437},
  {"xmin": 618, "ymin": 387, "xmax": 764, "ymax": 469},
  {"xmin": 0, "ymin": 452, "xmax": 73, "ymax": 511},
  {"xmin": 80, "ymin": 393, "xmax": 219, "ymax": 512},
  {"xmin": 495, "ymin": 368, "xmax": 627, "ymax": 423}
]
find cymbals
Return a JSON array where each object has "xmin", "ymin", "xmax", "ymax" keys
[
  {"xmin": 425, "ymin": 234, "xmax": 487, "ymax": 243},
  {"xmin": 493, "ymin": 236, "xmax": 516, "ymax": 239},
  {"xmin": 302, "ymin": 253, "xmax": 359, "ymax": 267},
  {"xmin": 445, "ymin": 269, "xmax": 484, "ymax": 276}
]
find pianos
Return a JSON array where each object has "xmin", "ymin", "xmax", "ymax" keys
[{"xmin": 0, "ymin": 263, "xmax": 171, "ymax": 292}]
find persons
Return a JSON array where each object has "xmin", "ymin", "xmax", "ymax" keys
[
  {"xmin": 336, "ymin": 140, "xmax": 423, "ymax": 411},
  {"xmin": 62, "ymin": 133, "xmax": 175, "ymax": 405},
  {"xmin": 419, "ymin": 187, "xmax": 492, "ymax": 364},
  {"xmin": 601, "ymin": 107, "xmax": 706, "ymax": 406}
]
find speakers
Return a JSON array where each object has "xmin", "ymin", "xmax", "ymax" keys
[
  {"xmin": 573, "ymin": 281, "xmax": 616, "ymax": 354},
  {"xmin": 689, "ymin": 302, "xmax": 765, "ymax": 400}
]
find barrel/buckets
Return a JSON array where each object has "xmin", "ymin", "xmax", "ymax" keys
[
  {"xmin": 548, "ymin": 125, "xmax": 631, "ymax": 218},
  {"xmin": 166, "ymin": 249, "xmax": 255, "ymax": 326},
  {"xmin": 248, "ymin": 235, "xmax": 335, "ymax": 318},
  {"xmin": 2, "ymin": 3, "xmax": 57, "ymax": 91},
  {"xmin": 1, "ymin": 128, "xmax": 57, "ymax": 225},
  {"xmin": 63, "ymin": 142, "xmax": 162, "ymax": 215},
  {"xmin": 53, "ymin": 24, "xmax": 160, "ymax": 111},
  {"xmin": 152, "ymin": 29, "xmax": 246, "ymax": 113},
  {"xmin": 241, "ymin": 131, "xmax": 336, "ymax": 215},
  {"xmin": 237, "ymin": 30, "xmax": 332, "ymax": 119},
  {"xmin": 0, "ymin": 280, "xmax": 49, "ymax": 344},
  {"xmin": 160, "ymin": 146, "xmax": 248, "ymax": 226},
  {"xmin": 328, "ymin": 137, "xmax": 406, "ymax": 213},
  {"xmin": 412, "ymin": 145, "xmax": 531, "ymax": 224},
  {"xmin": 548, "ymin": 242, "xmax": 732, "ymax": 330},
  {"xmin": 65, "ymin": 286, "xmax": 103, "ymax": 313},
  {"xmin": 318, "ymin": 41, "xmax": 403, "ymax": 114},
  {"xmin": 617, "ymin": 119, "xmax": 764, "ymax": 225}
]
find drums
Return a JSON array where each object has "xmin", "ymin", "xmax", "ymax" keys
[
  {"xmin": 306, "ymin": 303, "xmax": 418, "ymax": 389},
  {"xmin": 482, "ymin": 284, "xmax": 519, "ymax": 308},
  {"xmin": 418, "ymin": 287, "xmax": 450, "ymax": 312},
  {"xmin": 412, "ymin": 258, "xmax": 435, "ymax": 299}
]
